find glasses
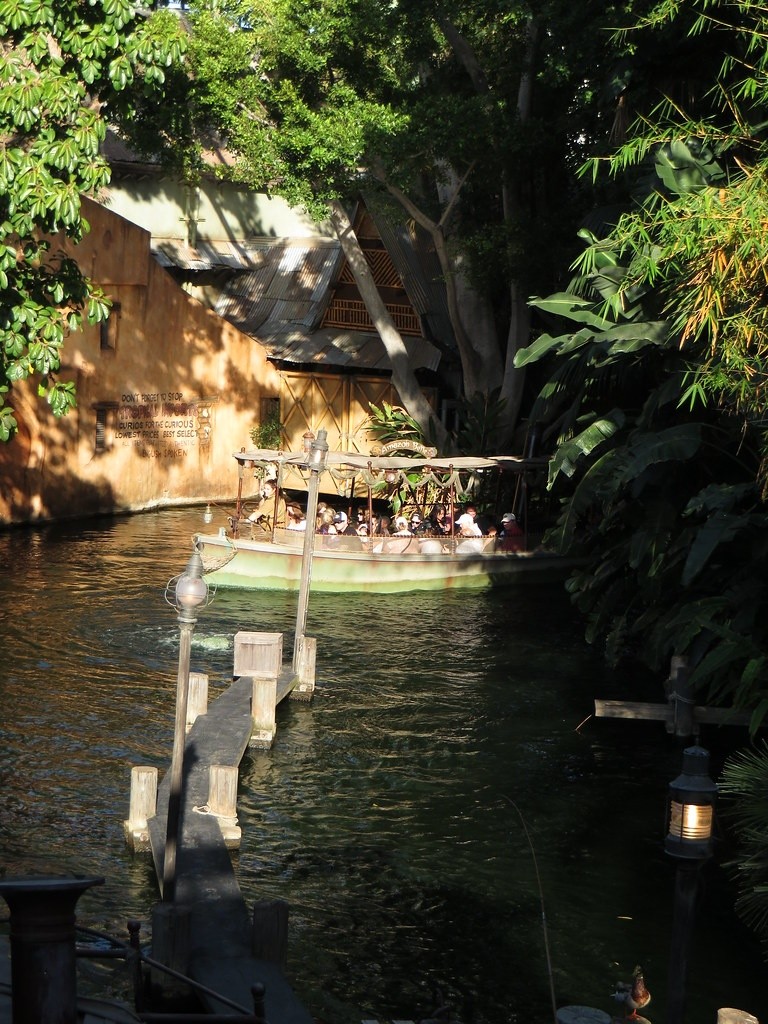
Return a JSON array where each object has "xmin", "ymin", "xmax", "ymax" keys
[
  {"xmin": 412, "ymin": 521, "xmax": 419, "ymax": 524},
  {"xmin": 334, "ymin": 520, "xmax": 345, "ymax": 524},
  {"xmin": 358, "ymin": 512, "xmax": 364, "ymax": 516}
]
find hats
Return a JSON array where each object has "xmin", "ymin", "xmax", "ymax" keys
[
  {"xmin": 333, "ymin": 511, "xmax": 348, "ymax": 521},
  {"xmin": 396, "ymin": 517, "xmax": 408, "ymax": 527},
  {"xmin": 501, "ymin": 512, "xmax": 516, "ymax": 523},
  {"xmin": 412, "ymin": 513, "xmax": 422, "ymax": 522},
  {"xmin": 455, "ymin": 514, "xmax": 474, "ymax": 524}
]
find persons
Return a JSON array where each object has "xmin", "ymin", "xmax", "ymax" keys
[
  {"xmin": 429, "ymin": 504, "xmax": 450, "ymax": 535},
  {"xmin": 392, "ymin": 517, "xmax": 417, "ymax": 554},
  {"xmin": 381, "ymin": 516, "xmax": 391, "ymax": 536},
  {"xmin": 286, "ymin": 502, "xmax": 363, "ymax": 550},
  {"xmin": 454, "ymin": 504, "xmax": 462, "ymax": 517},
  {"xmin": 455, "ymin": 503, "xmax": 483, "ymax": 535},
  {"xmin": 358, "ymin": 508, "xmax": 378, "ymax": 554},
  {"xmin": 411, "ymin": 514, "xmax": 421, "ymax": 528},
  {"xmin": 488, "ymin": 513, "xmax": 524, "ymax": 551},
  {"xmin": 246, "ymin": 479, "xmax": 289, "ymax": 530}
]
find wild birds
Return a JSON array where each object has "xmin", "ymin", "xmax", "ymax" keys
[{"xmin": 610, "ymin": 965, "xmax": 651, "ymax": 1020}]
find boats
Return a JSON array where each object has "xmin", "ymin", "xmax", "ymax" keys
[{"xmin": 191, "ymin": 432, "xmax": 595, "ymax": 596}]
